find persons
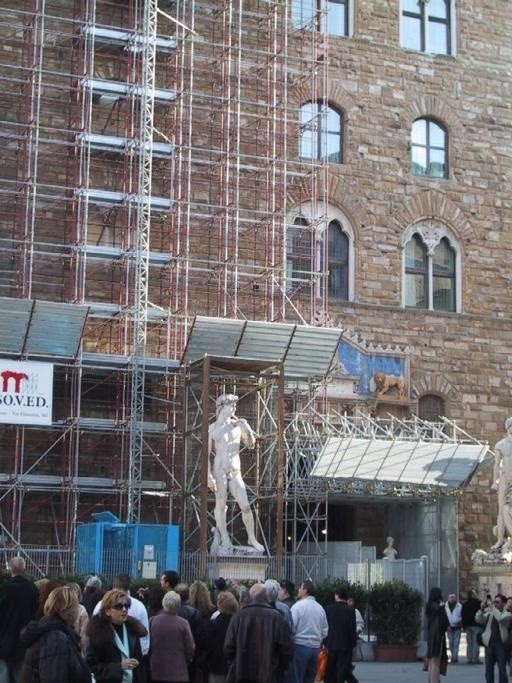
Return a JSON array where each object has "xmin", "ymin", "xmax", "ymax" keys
[
  {"xmin": 424, "ymin": 587, "xmax": 512, "ymax": 683},
  {"xmin": 382, "ymin": 536, "xmax": 399, "ymax": 559},
  {"xmin": 204, "ymin": 393, "xmax": 266, "ymax": 551},
  {"xmin": 1, "ymin": 557, "xmax": 364, "ymax": 683},
  {"xmin": 491, "ymin": 417, "xmax": 512, "ymax": 553}
]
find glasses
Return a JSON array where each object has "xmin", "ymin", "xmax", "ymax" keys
[{"xmin": 111, "ymin": 603, "xmax": 130, "ymax": 610}]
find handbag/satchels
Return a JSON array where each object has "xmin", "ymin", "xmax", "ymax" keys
[
  {"xmin": 477, "ymin": 632, "xmax": 485, "ymax": 646},
  {"xmin": 423, "ymin": 656, "xmax": 447, "ymax": 676}
]
